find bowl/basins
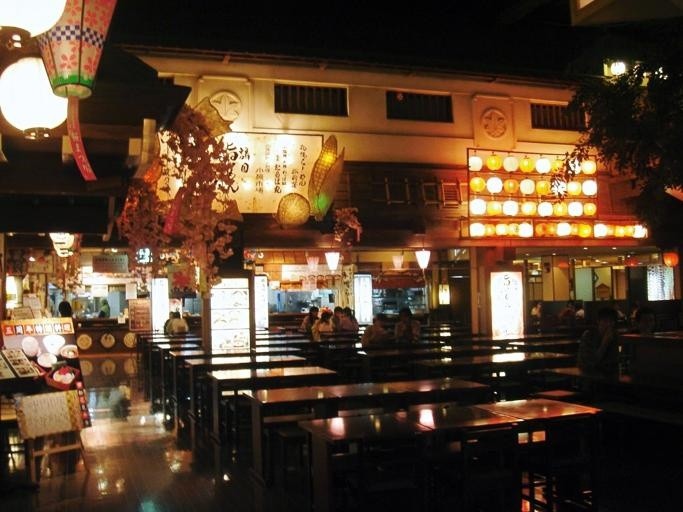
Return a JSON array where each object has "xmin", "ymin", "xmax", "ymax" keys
[{"xmin": 21, "ymin": 337, "xmax": 37, "ymax": 358}]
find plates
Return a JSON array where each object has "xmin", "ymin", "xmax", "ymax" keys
[
  {"xmin": 41, "ymin": 335, "xmax": 65, "ymax": 352},
  {"xmin": 36, "ymin": 354, "xmax": 57, "ymax": 369},
  {"xmin": 60, "ymin": 345, "xmax": 77, "ymax": 360}
]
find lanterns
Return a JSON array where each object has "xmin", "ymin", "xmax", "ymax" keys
[
  {"xmin": 49, "ymin": 232, "xmax": 75, "ymax": 257},
  {"xmin": 0, "ymin": 0, "xmax": 67, "ymax": 51},
  {"xmin": 134, "ymin": 247, "xmax": 156, "ymax": 266},
  {"xmin": 466, "ymin": 148, "xmax": 648, "ymax": 239},
  {"xmin": 661, "ymin": 252, "xmax": 679, "ymax": 268},
  {"xmin": 0, "ymin": 55, "xmax": 73, "ymax": 152},
  {"xmin": 34, "ymin": 2, "xmax": 130, "ymax": 97}
]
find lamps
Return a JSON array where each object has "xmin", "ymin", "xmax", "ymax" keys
[
  {"xmin": 305, "ymin": 237, "xmax": 340, "ymax": 274},
  {"xmin": 391, "ymin": 250, "xmax": 403, "ymax": 269},
  {"xmin": 607, "ymin": 60, "xmax": 627, "ymax": 77},
  {"xmin": 662, "ymin": 252, "xmax": 678, "ymax": 267},
  {"xmin": 1, "ymin": 0, "xmax": 69, "ymax": 50},
  {"xmin": 414, "ymin": 247, "xmax": 430, "ymax": 270},
  {"xmin": 467, "ymin": 148, "xmax": 648, "ymax": 238},
  {"xmin": 1, "ymin": 56, "xmax": 69, "ymax": 144}
]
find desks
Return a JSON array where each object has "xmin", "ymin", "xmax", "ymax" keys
[{"xmin": 139, "ymin": 322, "xmax": 681, "ymax": 470}]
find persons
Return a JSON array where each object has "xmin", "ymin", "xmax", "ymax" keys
[
  {"xmin": 59, "ymin": 302, "xmax": 77, "ymax": 336},
  {"xmin": 165, "ymin": 312, "xmax": 187, "ymax": 333},
  {"xmin": 361, "ymin": 312, "xmax": 387, "ymax": 346},
  {"xmin": 300, "ymin": 302, "xmax": 360, "ymax": 335},
  {"xmin": 529, "ymin": 301, "xmax": 657, "ymax": 390},
  {"xmin": 393, "ymin": 307, "xmax": 421, "ymax": 342},
  {"xmin": 96, "ymin": 298, "xmax": 111, "ymax": 318},
  {"xmin": 164, "ymin": 311, "xmax": 173, "ymax": 333}
]
